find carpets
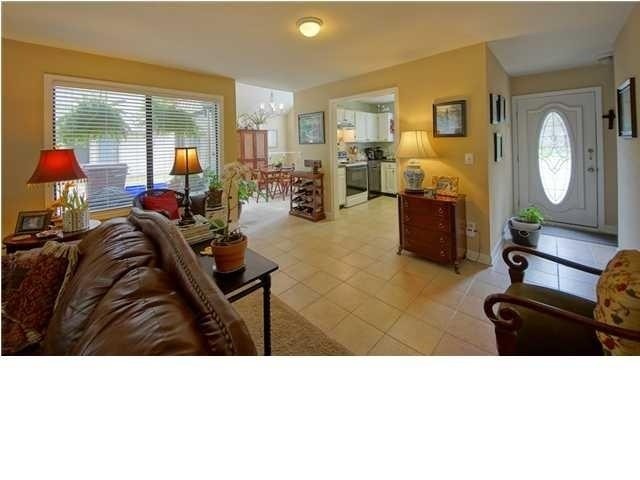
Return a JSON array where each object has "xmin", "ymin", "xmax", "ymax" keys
[{"xmin": 225, "ymin": 281, "xmax": 355, "ymax": 355}]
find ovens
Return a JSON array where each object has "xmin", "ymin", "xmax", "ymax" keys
[{"xmin": 344, "ymin": 164, "xmax": 368, "ymax": 208}]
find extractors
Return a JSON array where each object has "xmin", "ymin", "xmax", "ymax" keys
[{"xmin": 337, "ymin": 124, "xmax": 355, "ymax": 130}]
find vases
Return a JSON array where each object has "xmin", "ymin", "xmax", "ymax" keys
[{"xmin": 59, "ymin": 208, "xmax": 90, "ymax": 231}]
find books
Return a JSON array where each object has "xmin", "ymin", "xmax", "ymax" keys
[{"xmin": 171, "ymin": 213, "xmax": 216, "ymax": 247}]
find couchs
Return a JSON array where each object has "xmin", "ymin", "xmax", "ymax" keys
[{"xmin": 2, "ymin": 208, "xmax": 260, "ymax": 356}]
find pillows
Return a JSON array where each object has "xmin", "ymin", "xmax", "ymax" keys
[
  {"xmin": 140, "ymin": 192, "xmax": 178, "ymax": 219},
  {"xmin": 2, "ymin": 241, "xmax": 80, "ymax": 356},
  {"xmin": 593, "ymin": 245, "xmax": 640, "ymax": 356}
]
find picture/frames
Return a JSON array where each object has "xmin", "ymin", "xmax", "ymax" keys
[
  {"xmin": 615, "ymin": 75, "xmax": 636, "ymax": 140},
  {"xmin": 296, "ymin": 111, "xmax": 325, "ymax": 145},
  {"xmin": 497, "ymin": 94, "xmax": 507, "ymax": 123},
  {"xmin": 267, "ymin": 129, "xmax": 278, "ymax": 148},
  {"xmin": 494, "ymin": 132, "xmax": 504, "ymax": 162},
  {"xmin": 489, "ymin": 94, "xmax": 500, "ymax": 124},
  {"xmin": 430, "ymin": 100, "xmax": 468, "ymax": 137},
  {"xmin": 14, "ymin": 211, "xmax": 54, "ymax": 235},
  {"xmin": 431, "ymin": 174, "xmax": 458, "ymax": 197}
]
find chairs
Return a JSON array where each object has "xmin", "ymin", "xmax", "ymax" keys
[
  {"xmin": 133, "ymin": 187, "xmax": 187, "ymax": 221},
  {"xmin": 482, "ymin": 245, "xmax": 640, "ymax": 356},
  {"xmin": 247, "ymin": 161, "xmax": 296, "ymax": 203}
]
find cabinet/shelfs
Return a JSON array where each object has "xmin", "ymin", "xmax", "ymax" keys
[
  {"xmin": 236, "ymin": 129, "xmax": 268, "ymax": 169},
  {"xmin": 337, "ymin": 108, "xmax": 355, "ymax": 127},
  {"xmin": 381, "ymin": 161, "xmax": 396, "ymax": 196},
  {"xmin": 355, "ymin": 112, "xmax": 377, "ymax": 141},
  {"xmin": 289, "ymin": 170, "xmax": 325, "ymax": 221},
  {"xmin": 377, "ymin": 113, "xmax": 395, "ymax": 142},
  {"xmin": 395, "ymin": 189, "xmax": 470, "ymax": 276},
  {"xmin": 337, "ymin": 166, "xmax": 347, "ymax": 206}
]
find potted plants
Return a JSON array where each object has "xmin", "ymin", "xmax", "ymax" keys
[
  {"xmin": 510, "ymin": 207, "xmax": 545, "ymax": 230},
  {"xmin": 205, "ymin": 172, "xmax": 225, "ymax": 208},
  {"xmin": 239, "ymin": 108, "xmax": 273, "ymax": 129},
  {"xmin": 204, "ymin": 162, "xmax": 266, "ymax": 275}
]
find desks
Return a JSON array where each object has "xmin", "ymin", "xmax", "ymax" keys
[
  {"xmin": 205, "ymin": 207, "xmax": 227, "ymax": 229},
  {"xmin": 2, "ymin": 219, "xmax": 102, "ymax": 255},
  {"xmin": 190, "ymin": 235, "xmax": 285, "ymax": 355}
]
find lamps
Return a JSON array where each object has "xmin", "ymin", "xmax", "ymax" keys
[
  {"xmin": 396, "ymin": 131, "xmax": 434, "ymax": 191},
  {"xmin": 258, "ymin": 91, "xmax": 288, "ymax": 116},
  {"xmin": 168, "ymin": 145, "xmax": 204, "ymax": 225},
  {"xmin": 296, "ymin": 16, "xmax": 323, "ymax": 39},
  {"xmin": 25, "ymin": 148, "xmax": 89, "ymax": 210}
]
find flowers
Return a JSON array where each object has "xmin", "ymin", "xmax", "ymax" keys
[{"xmin": 51, "ymin": 180, "xmax": 91, "ymax": 213}]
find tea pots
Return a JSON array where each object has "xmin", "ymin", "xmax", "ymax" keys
[{"xmin": 350, "ymin": 146, "xmax": 358, "ymax": 154}]
